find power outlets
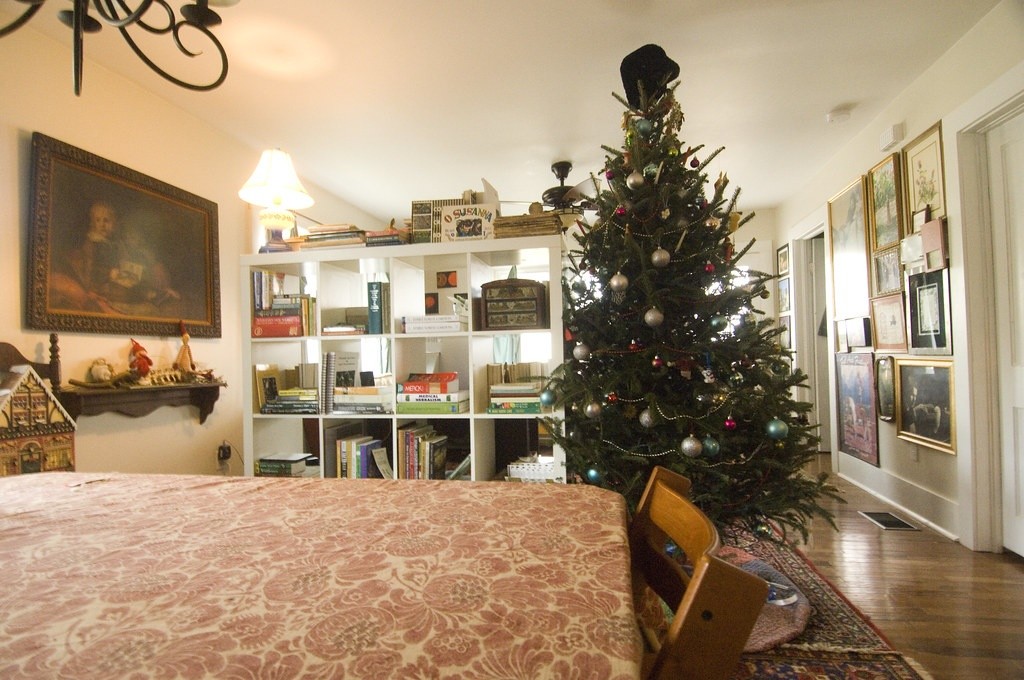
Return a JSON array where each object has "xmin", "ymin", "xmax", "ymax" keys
[{"xmin": 215, "ymin": 450, "xmax": 227, "ymax": 471}]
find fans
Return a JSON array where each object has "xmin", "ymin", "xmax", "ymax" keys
[{"xmin": 498, "ymin": 161, "xmax": 605, "ymax": 210}]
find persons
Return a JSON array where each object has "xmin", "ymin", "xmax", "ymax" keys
[{"xmin": 901, "ymin": 375, "xmax": 929, "ymax": 434}]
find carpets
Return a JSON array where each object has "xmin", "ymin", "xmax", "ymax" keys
[{"xmin": 731, "ymin": 517, "xmax": 937, "ymax": 680}]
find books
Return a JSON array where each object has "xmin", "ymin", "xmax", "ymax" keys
[
  {"xmin": 299, "ymin": 224, "xmax": 409, "ymax": 249},
  {"xmin": 401, "ymin": 316, "xmax": 467, "ymax": 333},
  {"xmin": 322, "ymin": 352, "xmax": 393, "ymax": 414},
  {"xmin": 324, "ymin": 421, "xmax": 381, "ymax": 478},
  {"xmin": 254, "ymin": 452, "xmax": 313, "ymax": 474},
  {"xmin": 251, "ymin": 269, "xmax": 316, "ymax": 338},
  {"xmin": 322, "ymin": 282, "xmax": 390, "ymax": 336},
  {"xmin": 255, "ymin": 363, "xmax": 319, "ymax": 414},
  {"xmin": 487, "ymin": 362, "xmax": 543, "ymax": 414},
  {"xmin": 397, "ymin": 420, "xmax": 470, "ymax": 480},
  {"xmin": 396, "ymin": 373, "xmax": 470, "ymax": 414}
]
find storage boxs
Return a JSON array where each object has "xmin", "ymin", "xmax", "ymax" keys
[{"xmin": 440, "ymin": 205, "xmax": 499, "ymax": 242}]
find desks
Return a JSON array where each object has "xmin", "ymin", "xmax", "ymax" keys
[{"xmin": 1, "ymin": 472, "xmax": 641, "ymax": 680}]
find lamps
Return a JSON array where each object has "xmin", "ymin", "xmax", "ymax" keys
[
  {"xmin": 239, "ymin": 147, "xmax": 313, "ymax": 254},
  {"xmin": 0, "ymin": 0, "xmax": 243, "ymax": 97},
  {"xmin": 548, "ymin": 207, "xmax": 585, "ymax": 233}
]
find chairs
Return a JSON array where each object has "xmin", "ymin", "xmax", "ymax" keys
[{"xmin": 623, "ymin": 463, "xmax": 767, "ymax": 680}]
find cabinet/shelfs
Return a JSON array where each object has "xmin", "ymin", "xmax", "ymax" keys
[{"xmin": 240, "ymin": 236, "xmax": 566, "ymax": 482}]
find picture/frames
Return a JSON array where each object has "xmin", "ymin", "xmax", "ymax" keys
[
  {"xmin": 775, "ymin": 243, "xmax": 790, "ymax": 277},
  {"xmin": 871, "ymin": 292, "xmax": 909, "ymax": 355},
  {"xmin": 871, "ymin": 246, "xmax": 907, "ymax": 299},
  {"xmin": 901, "ymin": 120, "xmax": 949, "ymax": 236},
  {"xmin": 28, "ymin": 131, "xmax": 228, "ymax": 336},
  {"xmin": 894, "ymin": 358, "xmax": 957, "ymax": 456},
  {"xmin": 834, "ymin": 351, "xmax": 880, "ymax": 467},
  {"xmin": 780, "ymin": 356, "xmax": 793, "ymax": 377},
  {"xmin": 777, "ymin": 278, "xmax": 791, "ymax": 313},
  {"xmin": 825, "ymin": 175, "xmax": 872, "ymax": 320},
  {"xmin": 779, "ymin": 315, "xmax": 791, "ymax": 349},
  {"xmin": 910, "ymin": 274, "xmax": 947, "ymax": 349},
  {"xmin": 868, "ymin": 153, "xmax": 904, "ymax": 253},
  {"xmin": 875, "ymin": 356, "xmax": 894, "ymax": 421}
]
